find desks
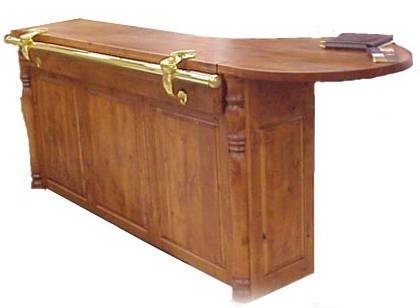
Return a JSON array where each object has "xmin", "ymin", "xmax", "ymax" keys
[{"xmin": 4, "ymin": 17, "xmax": 414, "ymax": 304}]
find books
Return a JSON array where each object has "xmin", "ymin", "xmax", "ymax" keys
[
  {"xmin": 319, "ymin": 32, "xmax": 396, "ymax": 52},
  {"xmin": 368, "ymin": 47, "xmax": 398, "ymax": 63}
]
